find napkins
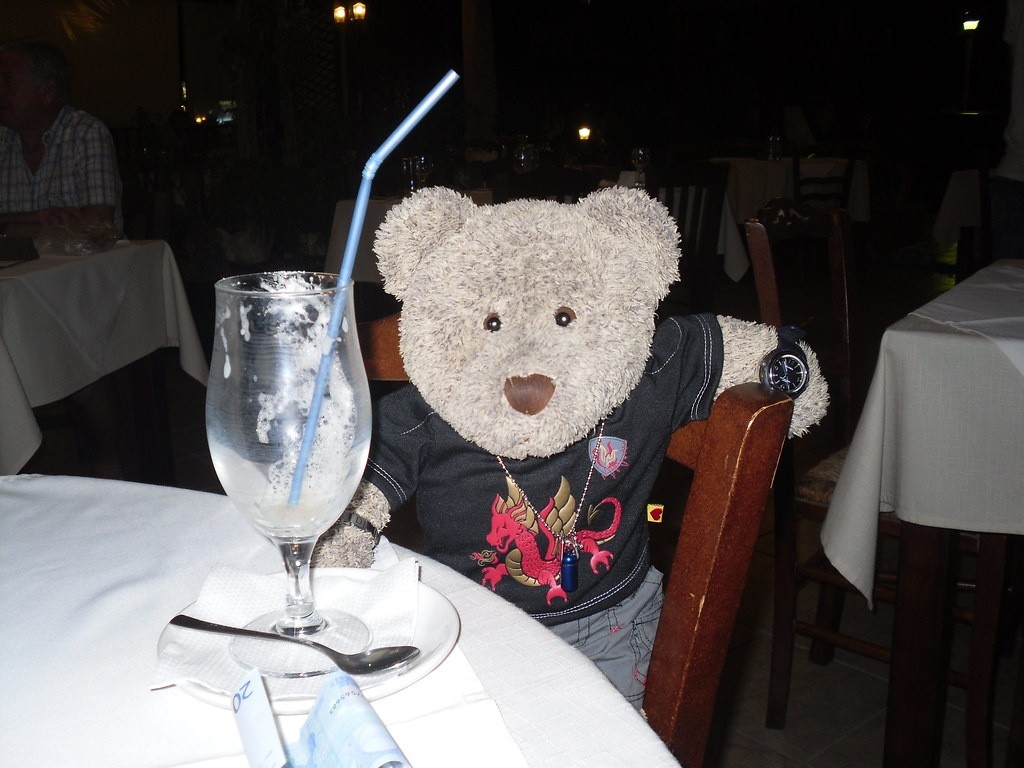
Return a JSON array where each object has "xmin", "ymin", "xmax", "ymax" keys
[{"xmin": 158, "ymin": 555, "xmax": 420, "ymax": 699}]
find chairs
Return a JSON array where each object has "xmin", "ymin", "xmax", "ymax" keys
[
  {"xmin": 791, "ymin": 145, "xmax": 857, "ymax": 210},
  {"xmin": 361, "ymin": 311, "xmax": 795, "ymax": 768},
  {"xmin": 111, "ymin": 125, "xmax": 162, "ymax": 237},
  {"xmin": 744, "ymin": 208, "xmax": 1014, "ymax": 767}
]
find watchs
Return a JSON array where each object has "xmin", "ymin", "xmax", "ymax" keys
[{"xmin": 760, "ymin": 325, "xmax": 812, "ymax": 403}]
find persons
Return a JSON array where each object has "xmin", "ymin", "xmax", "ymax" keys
[{"xmin": 0, "ymin": 42, "xmax": 126, "ymax": 238}]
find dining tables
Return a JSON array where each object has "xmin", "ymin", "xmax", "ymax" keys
[{"xmin": 3, "ymin": 471, "xmax": 687, "ymax": 766}]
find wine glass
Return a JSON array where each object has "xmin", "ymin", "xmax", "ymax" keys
[{"xmin": 203, "ymin": 267, "xmax": 375, "ymax": 683}]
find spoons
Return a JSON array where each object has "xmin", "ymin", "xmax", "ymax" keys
[{"xmin": 167, "ymin": 611, "xmax": 420, "ymax": 677}]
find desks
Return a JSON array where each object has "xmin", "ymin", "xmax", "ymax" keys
[
  {"xmin": 820, "ymin": 259, "xmax": 1024, "ymax": 768},
  {"xmin": 0, "ymin": 237, "xmax": 210, "ymax": 485},
  {"xmin": 327, "ymin": 156, "xmax": 991, "ymax": 307}
]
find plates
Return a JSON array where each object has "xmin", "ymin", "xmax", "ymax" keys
[{"xmin": 155, "ymin": 571, "xmax": 462, "ymax": 714}]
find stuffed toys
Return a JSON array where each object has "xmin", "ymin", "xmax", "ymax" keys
[{"xmin": 310, "ymin": 187, "xmax": 831, "ymax": 722}]
